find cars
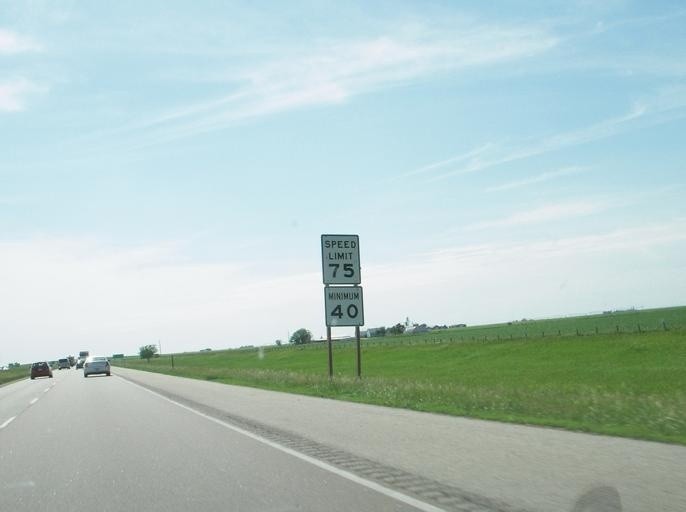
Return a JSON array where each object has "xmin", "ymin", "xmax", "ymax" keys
[
  {"xmin": 29, "ymin": 362, "xmax": 53, "ymax": 379},
  {"xmin": 58, "ymin": 351, "xmax": 111, "ymax": 378}
]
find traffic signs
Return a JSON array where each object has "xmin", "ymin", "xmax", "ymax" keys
[
  {"xmin": 324, "ymin": 287, "xmax": 364, "ymax": 326},
  {"xmin": 321, "ymin": 234, "xmax": 360, "ymax": 284}
]
